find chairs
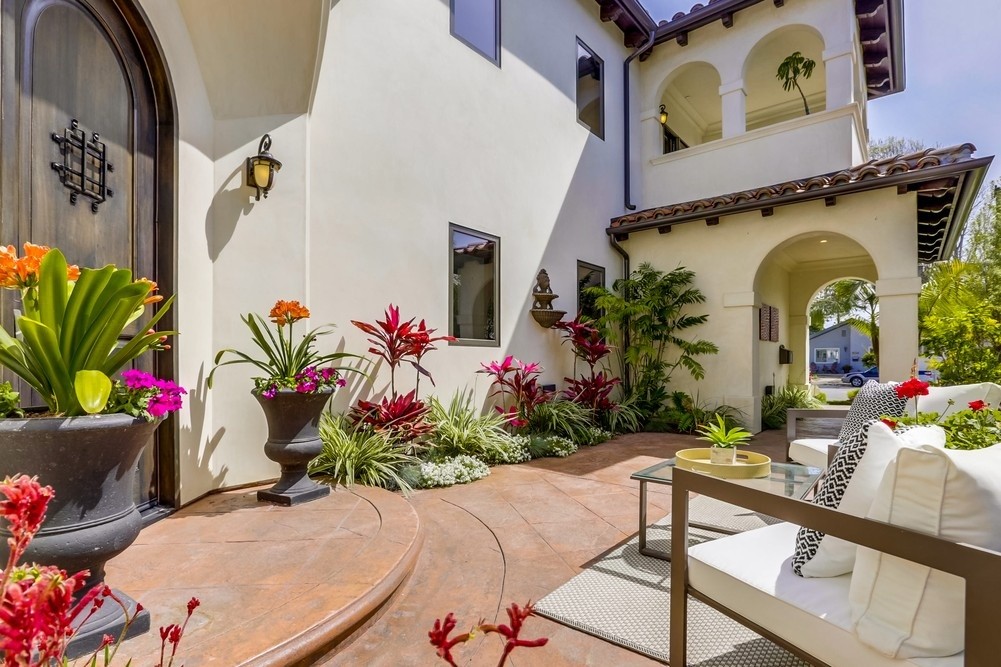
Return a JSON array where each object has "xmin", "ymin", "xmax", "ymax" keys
[{"xmin": 670, "ymin": 380, "xmax": 1001, "ymax": 667}]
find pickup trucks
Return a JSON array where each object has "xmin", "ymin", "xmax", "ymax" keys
[{"xmin": 840, "ymin": 366, "xmax": 934, "ymax": 387}]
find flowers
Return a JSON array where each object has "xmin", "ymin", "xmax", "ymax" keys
[
  {"xmin": 0, "ymin": 242, "xmax": 187, "ymax": 425},
  {"xmin": 208, "ymin": 299, "xmax": 375, "ymax": 399}
]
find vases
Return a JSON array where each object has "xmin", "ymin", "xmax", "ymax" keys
[
  {"xmin": 0, "ymin": 405, "xmax": 169, "ymax": 657},
  {"xmin": 251, "ymin": 387, "xmax": 336, "ymax": 505}
]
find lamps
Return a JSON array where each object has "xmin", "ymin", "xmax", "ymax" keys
[
  {"xmin": 245, "ymin": 133, "xmax": 281, "ymax": 200},
  {"xmin": 659, "ymin": 104, "xmax": 668, "ymax": 126}
]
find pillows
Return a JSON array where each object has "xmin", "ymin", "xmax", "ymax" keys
[
  {"xmin": 837, "ymin": 379, "xmax": 918, "ymax": 442},
  {"xmin": 792, "ymin": 423, "xmax": 945, "ymax": 578}
]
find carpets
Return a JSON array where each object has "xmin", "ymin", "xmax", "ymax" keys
[{"xmin": 531, "ymin": 477, "xmax": 818, "ymax": 667}]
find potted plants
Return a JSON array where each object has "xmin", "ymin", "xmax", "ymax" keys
[{"xmin": 695, "ymin": 413, "xmax": 754, "ymax": 464}]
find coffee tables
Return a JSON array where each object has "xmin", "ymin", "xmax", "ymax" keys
[{"xmin": 630, "ymin": 457, "xmax": 824, "ymax": 562}]
